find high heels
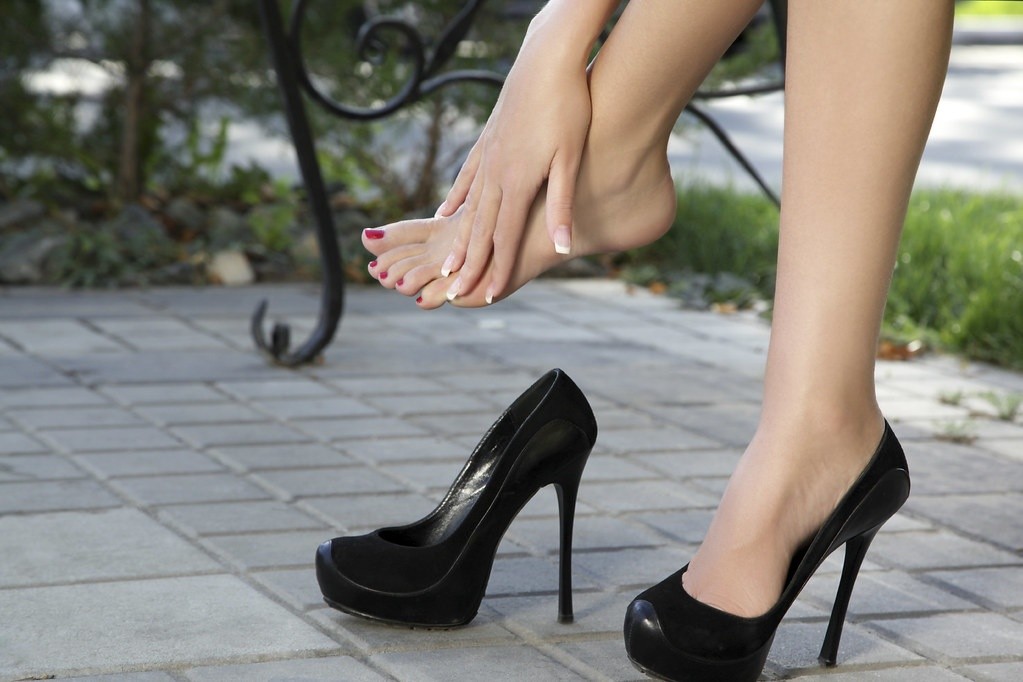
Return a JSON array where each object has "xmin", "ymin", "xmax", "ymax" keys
[
  {"xmin": 315, "ymin": 367, "xmax": 598, "ymax": 630},
  {"xmin": 624, "ymin": 417, "xmax": 910, "ymax": 682}
]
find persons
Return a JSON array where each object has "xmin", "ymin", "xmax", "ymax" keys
[{"xmin": 362, "ymin": 0, "xmax": 954, "ymax": 682}]
